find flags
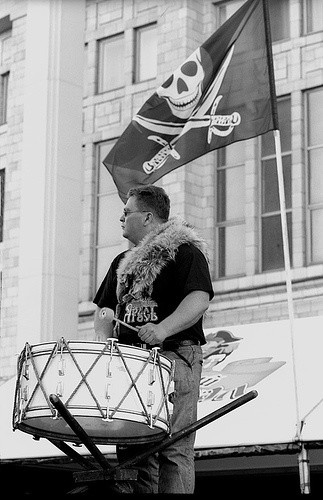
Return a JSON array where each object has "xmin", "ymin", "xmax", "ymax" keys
[{"xmin": 100, "ymin": 0, "xmax": 279, "ymax": 208}]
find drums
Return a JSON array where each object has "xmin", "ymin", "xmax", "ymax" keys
[{"xmin": 11, "ymin": 338, "xmax": 175, "ymax": 450}]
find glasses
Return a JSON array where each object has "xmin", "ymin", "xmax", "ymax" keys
[{"xmin": 124, "ymin": 210, "xmax": 148, "ymax": 216}]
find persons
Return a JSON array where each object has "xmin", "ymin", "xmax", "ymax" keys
[{"xmin": 90, "ymin": 185, "xmax": 216, "ymax": 496}]
